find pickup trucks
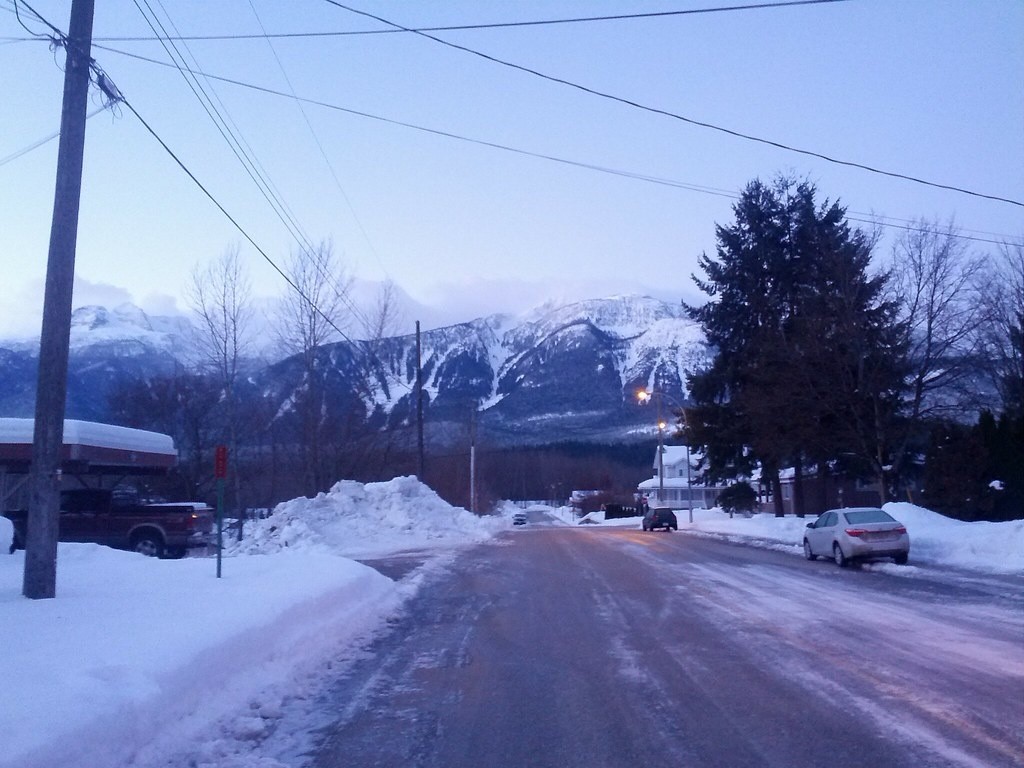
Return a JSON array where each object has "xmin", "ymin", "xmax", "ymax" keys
[{"xmin": 0, "ymin": 488, "xmax": 215, "ymax": 560}]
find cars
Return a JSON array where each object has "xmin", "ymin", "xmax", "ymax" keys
[
  {"xmin": 802, "ymin": 507, "xmax": 911, "ymax": 568},
  {"xmin": 642, "ymin": 507, "xmax": 677, "ymax": 533}
]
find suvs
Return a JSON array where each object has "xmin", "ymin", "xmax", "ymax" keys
[{"xmin": 512, "ymin": 513, "xmax": 527, "ymax": 526}]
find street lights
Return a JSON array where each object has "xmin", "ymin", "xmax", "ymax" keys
[
  {"xmin": 637, "ymin": 390, "xmax": 694, "ymax": 523},
  {"xmin": 656, "ymin": 417, "xmax": 665, "ymax": 505}
]
